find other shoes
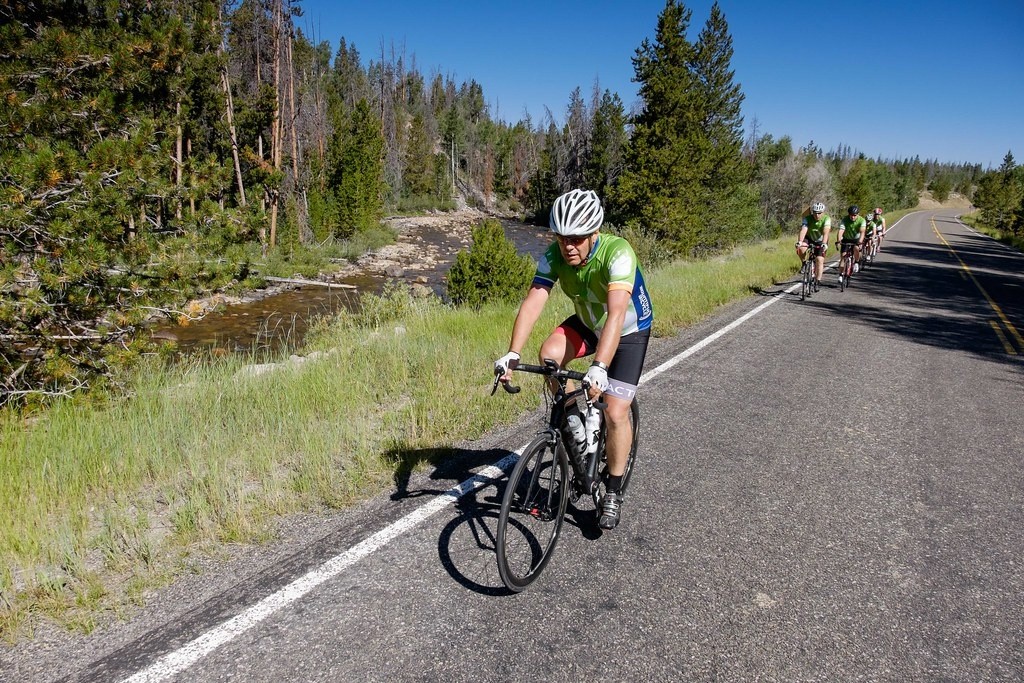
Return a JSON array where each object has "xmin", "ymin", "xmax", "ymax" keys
[
  {"xmin": 838, "ymin": 276, "xmax": 842, "ymax": 283},
  {"xmin": 876, "ymin": 246, "xmax": 881, "ymax": 252},
  {"xmin": 866, "ymin": 254, "xmax": 870, "ymax": 261},
  {"xmin": 853, "ymin": 262, "xmax": 859, "ymax": 273}
]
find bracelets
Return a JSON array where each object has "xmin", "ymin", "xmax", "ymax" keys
[{"xmin": 590, "ymin": 361, "xmax": 609, "ymax": 372}]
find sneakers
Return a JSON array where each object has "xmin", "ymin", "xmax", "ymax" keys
[
  {"xmin": 580, "ymin": 412, "xmax": 585, "ymax": 425},
  {"xmin": 801, "ymin": 264, "xmax": 806, "ymax": 274},
  {"xmin": 599, "ymin": 489, "xmax": 622, "ymax": 530},
  {"xmin": 814, "ymin": 282, "xmax": 820, "ymax": 292}
]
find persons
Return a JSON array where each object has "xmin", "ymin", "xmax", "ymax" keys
[
  {"xmin": 873, "ymin": 208, "xmax": 886, "ymax": 252},
  {"xmin": 493, "ymin": 187, "xmax": 656, "ymax": 531},
  {"xmin": 795, "ymin": 202, "xmax": 831, "ymax": 293},
  {"xmin": 836, "ymin": 205, "xmax": 867, "ymax": 283},
  {"xmin": 861, "ymin": 212, "xmax": 877, "ymax": 261}
]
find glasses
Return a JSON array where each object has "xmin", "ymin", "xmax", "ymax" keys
[
  {"xmin": 554, "ymin": 231, "xmax": 595, "ymax": 246},
  {"xmin": 849, "ymin": 214, "xmax": 856, "ymax": 216},
  {"xmin": 813, "ymin": 212, "xmax": 822, "ymax": 215}
]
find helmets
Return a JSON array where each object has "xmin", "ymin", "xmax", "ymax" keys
[
  {"xmin": 865, "ymin": 214, "xmax": 872, "ymax": 222},
  {"xmin": 848, "ymin": 206, "xmax": 859, "ymax": 215},
  {"xmin": 549, "ymin": 188, "xmax": 604, "ymax": 237},
  {"xmin": 874, "ymin": 208, "xmax": 882, "ymax": 215},
  {"xmin": 812, "ymin": 203, "xmax": 826, "ymax": 212}
]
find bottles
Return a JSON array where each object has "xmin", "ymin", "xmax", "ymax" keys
[
  {"xmin": 568, "ymin": 415, "xmax": 588, "ymax": 456},
  {"xmin": 585, "ymin": 406, "xmax": 600, "ymax": 453}
]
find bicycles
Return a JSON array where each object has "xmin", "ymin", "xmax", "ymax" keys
[
  {"xmin": 491, "ymin": 357, "xmax": 641, "ymax": 594},
  {"xmin": 835, "ymin": 232, "xmax": 887, "ymax": 292},
  {"xmin": 798, "ymin": 244, "xmax": 818, "ymax": 301}
]
